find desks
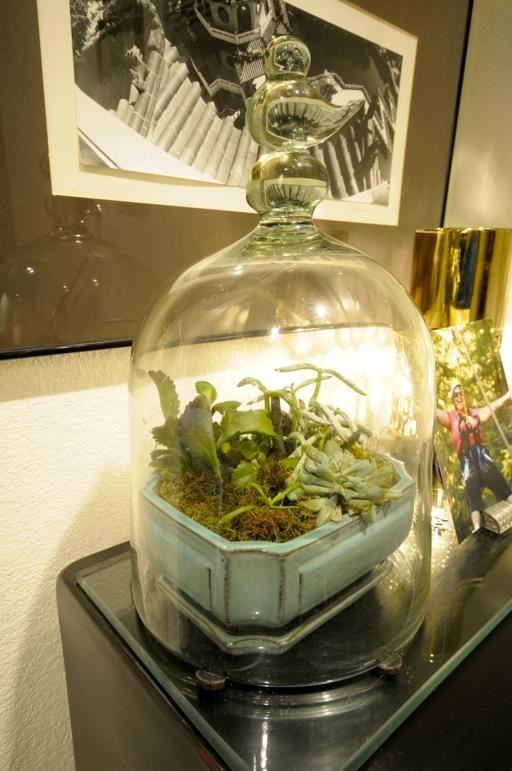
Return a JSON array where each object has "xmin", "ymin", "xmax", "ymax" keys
[{"xmin": 55, "ymin": 479, "xmax": 512, "ymax": 771}]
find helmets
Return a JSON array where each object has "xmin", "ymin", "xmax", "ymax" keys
[{"xmin": 448, "ymin": 382, "xmax": 462, "ymax": 399}]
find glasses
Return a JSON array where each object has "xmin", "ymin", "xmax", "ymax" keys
[{"xmin": 453, "ymin": 391, "xmax": 463, "ymax": 397}]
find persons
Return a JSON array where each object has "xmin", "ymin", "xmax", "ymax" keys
[{"xmin": 434, "ymin": 381, "xmax": 512, "ymax": 533}]
